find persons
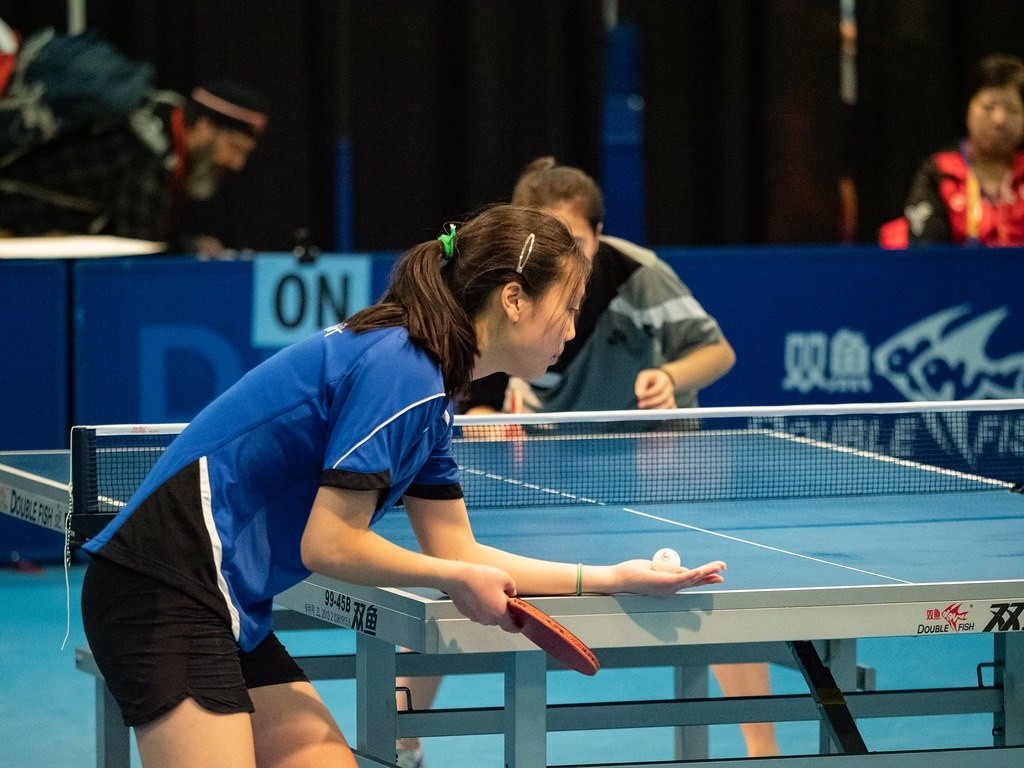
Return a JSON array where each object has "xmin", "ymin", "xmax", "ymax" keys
[
  {"xmin": 459, "ymin": 160, "xmax": 734, "ymax": 437},
  {"xmin": 81, "ymin": 205, "xmax": 726, "ymax": 768},
  {"xmin": 131, "ymin": 79, "xmax": 315, "ymax": 259},
  {"xmin": 902, "ymin": 56, "xmax": 1024, "ymax": 247}
]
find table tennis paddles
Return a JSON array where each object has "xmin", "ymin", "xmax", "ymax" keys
[
  {"xmin": 511, "ymin": 389, "xmax": 524, "ymax": 465},
  {"xmin": 504, "ymin": 591, "xmax": 600, "ymax": 676}
]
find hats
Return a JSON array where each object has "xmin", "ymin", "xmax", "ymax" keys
[{"xmin": 184, "ymin": 76, "xmax": 267, "ymax": 138}]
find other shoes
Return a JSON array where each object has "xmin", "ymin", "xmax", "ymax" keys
[{"xmin": 397, "ymin": 747, "xmax": 425, "ymax": 768}]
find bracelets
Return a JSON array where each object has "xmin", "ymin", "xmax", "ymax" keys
[
  {"xmin": 658, "ymin": 366, "xmax": 677, "ymax": 388},
  {"xmin": 577, "ymin": 563, "xmax": 584, "ymax": 596}
]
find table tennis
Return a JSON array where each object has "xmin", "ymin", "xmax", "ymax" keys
[{"xmin": 652, "ymin": 548, "xmax": 681, "ymax": 575}]
